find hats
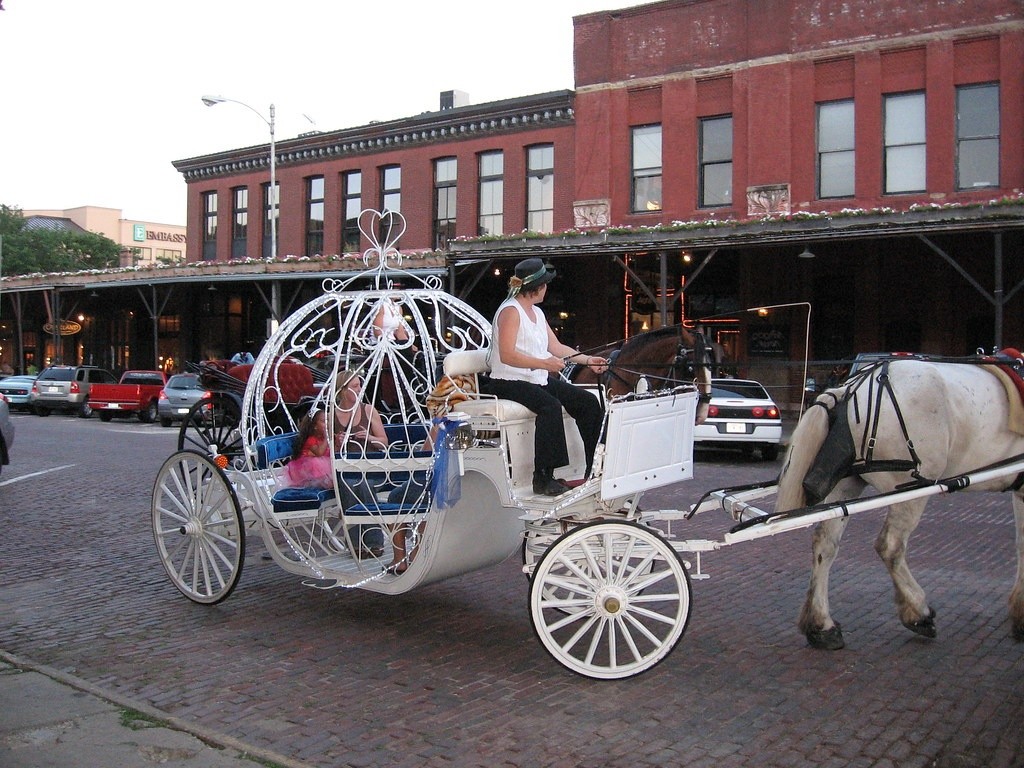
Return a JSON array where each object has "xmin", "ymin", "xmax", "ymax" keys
[{"xmin": 504, "ymin": 258, "xmax": 557, "ymax": 301}]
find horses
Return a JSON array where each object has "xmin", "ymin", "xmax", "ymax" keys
[
  {"xmin": 771, "ymin": 354, "xmax": 1024, "ymax": 651},
  {"xmin": 549, "ymin": 324, "xmax": 721, "ymax": 426}
]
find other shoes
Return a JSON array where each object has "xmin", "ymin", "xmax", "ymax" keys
[
  {"xmin": 351, "ymin": 547, "xmax": 370, "ymax": 560},
  {"xmin": 532, "ymin": 472, "xmax": 573, "ymax": 496},
  {"xmin": 368, "ymin": 544, "xmax": 384, "ymax": 559}
]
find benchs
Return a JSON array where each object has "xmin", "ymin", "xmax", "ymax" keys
[
  {"xmin": 260, "ymin": 410, "xmax": 465, "ymax": 525},
  {"xmin": 444, "ymin": 349, "xmax": 605, "ymax": 489}
]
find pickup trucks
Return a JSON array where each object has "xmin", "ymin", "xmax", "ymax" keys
[{"xmin": 87, "ymin": 369, "xmax": 171, "ymax": 424}]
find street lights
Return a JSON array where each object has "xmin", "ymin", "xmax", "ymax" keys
[{"xmin": 202, "ymin": 93, "xmax": 277, "ymax": 339}]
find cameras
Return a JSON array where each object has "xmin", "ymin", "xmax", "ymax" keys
[{"xmin": 348, "ymin": 433, "xmax": 355, "ymax": 440}]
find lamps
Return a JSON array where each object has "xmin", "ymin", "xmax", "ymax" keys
[
  {"xmin": 207, "ymin": 281, "xmax": 218, "ymax": 289},
  {"xmin": 798, "ymin": 245, "xmax": 816, "ymax": 257},
  {"xmin": 401, "ymin": 311, "xmax": 410, "ymax": 322},
  {"xmin": 544, "ymin": 256, "xmax": 554, "ymax": 269},
  {"xmin": 645, "ymin": 187, "xmax": 661, "ymax": 210},
  {"xmin": 90, "ymin": 290, "xmax": 97, "ymax": 300}
]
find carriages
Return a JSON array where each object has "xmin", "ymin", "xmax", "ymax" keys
[{"xmin": 151, "ymin": 209, "xmax": 1024, "ymax": 682}]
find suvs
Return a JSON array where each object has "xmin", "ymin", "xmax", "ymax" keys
[{"xmin": 31, "ymin": 365, "xmax": 119, "ymax": 419}]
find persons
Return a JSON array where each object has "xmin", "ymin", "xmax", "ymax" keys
[
  {"xmin": 2, "ymin": 361, "xmax": 39, "ymax": 376},
  {"xmin": 372, "ymin": 280, "xmax": 419, "ymax": 357},
  {"xmin": 488, "ymin": 258, "xmax": 608, "ymax": 496},
  {"xmin": 283, "ymin": 370, "xmax": 387, "ymax": 560},
  {"xmin": 381, "ymin": 373, "xmax": 477, "ymax": 575}
]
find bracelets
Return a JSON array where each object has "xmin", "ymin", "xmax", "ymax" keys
[{"xmin": 586, "ymin": 356, "xmax": 592, "ymax": 368}]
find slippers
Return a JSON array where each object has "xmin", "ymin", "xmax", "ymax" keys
[{"xmin": 382, "ymin": 562, "xmax": 410, "ymax": 575}]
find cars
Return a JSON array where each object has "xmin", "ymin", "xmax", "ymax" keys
[
  {"xmin": 0, "ymin": 375, "xmax": 39, "ymax": 415},
  {"xmin": 158, "ymin": 374, "xmax": 216, "ymax": 429},
  {"xmin": 306, "ymin": 355, "xmax": 372, "ymax": 374},
  {"xmin": 850, "ymin": 353, "xmax": 939, "ymax": 379},
  {"xmin": 693, "ymin": 379, "xmax": 782, "ymax": 463},
  {"xmin": 0, "ymin": 391, "xmax": 16, "ymax": 476}
]
